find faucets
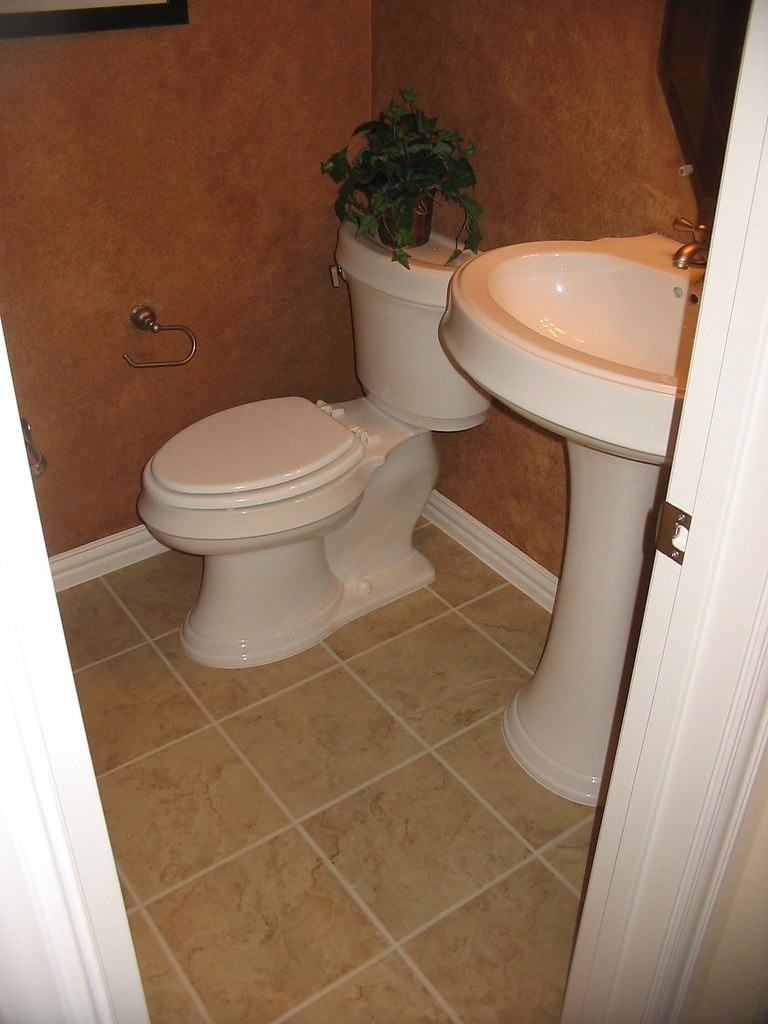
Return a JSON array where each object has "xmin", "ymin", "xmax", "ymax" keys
[{"xmin": 670, "ymin": 217, "xmax": 709, "ymax": 269}]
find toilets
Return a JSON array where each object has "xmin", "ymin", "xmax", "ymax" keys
[{"xmin": 137, "ymin": 217, "xmax": 485, "ymax": 669}]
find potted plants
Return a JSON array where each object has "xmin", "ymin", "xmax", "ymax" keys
[{"xmin": 319, "ymin": 84, "xmax": 486, "ymax": 266}]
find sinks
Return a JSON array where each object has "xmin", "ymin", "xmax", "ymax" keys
[{"xmin": 437, "ymin": 232, "xmax": 706, "ymax": 469}]
